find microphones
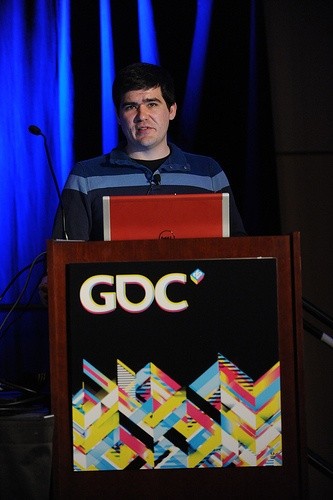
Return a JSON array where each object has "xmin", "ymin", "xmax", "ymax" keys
[{"xmin": 29, "ymin": 125, "xmax": 69, "ymax": 240}]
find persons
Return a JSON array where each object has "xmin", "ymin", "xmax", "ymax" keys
[{"xmin": 49, "ymin": 60, "xmax": 243, "ymax": 241}]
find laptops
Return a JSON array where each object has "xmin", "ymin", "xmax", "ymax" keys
[{"xmin": 103, "ymin": 193, "xmax": 230, "ymax": 241}]
